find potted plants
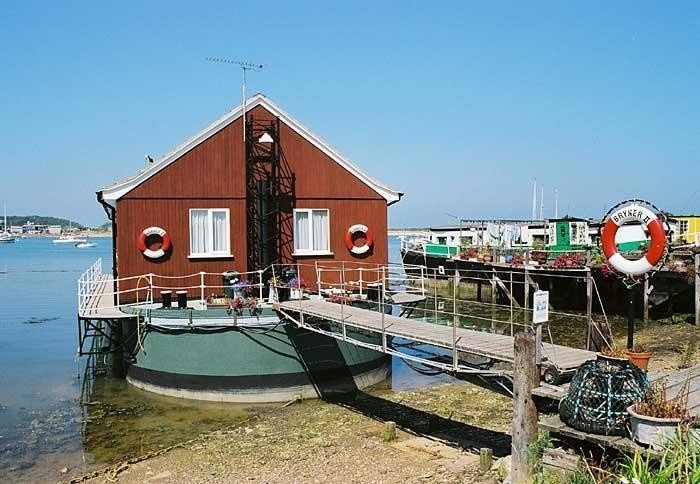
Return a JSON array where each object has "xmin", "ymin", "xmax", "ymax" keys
[{"xmin": 267, "ymin": 277, "xmax": 286, "ymax": 303}]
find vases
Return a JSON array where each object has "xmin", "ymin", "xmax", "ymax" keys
[
  {"xmin": 234, "ymin": 291, "xmax": 246, "ymax": 300},
  {"xmin": 290, "ymin": 287, "xmax": 303, "ymax": 299},
  {"xmin": 627, "ymin": 404, "xmax": 686, "ymax": 449},
  {"xmin": 627, "ymin": 350, "xmax": 651, "ymax": 372}
]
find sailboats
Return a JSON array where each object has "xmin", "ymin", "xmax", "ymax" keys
[
  {"xmin": 53, "ymin": 218, "xmax": 89, "ymax": 245},
  {"xmin": 0, "ymin": 204, "xmax": 19, "ymax": 243}
]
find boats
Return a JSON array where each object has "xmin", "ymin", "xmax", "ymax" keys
[
  {"xmin": 75, "ymin": 242, "xmax": 100, "ymax": 249},
  {"xmin": 399, "ymin": 217, "xmax": 681, "ymax": 308}
]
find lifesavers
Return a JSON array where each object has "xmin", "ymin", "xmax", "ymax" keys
[
  {"xmin": 600, "ymin": 207, "xmax": 667, "ymax": 277},
  {"xmin": 344, "ymin": 225, "xmax": 374, "ymax": 255}
]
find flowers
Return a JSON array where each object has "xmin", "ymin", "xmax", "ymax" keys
[
  {"xmin": 287, "ymin": 277, "xmax": 307, "ymax": 289},
  {"xmin": 231, "ymin": 280, "xmax": 253, "ymax": 293}
]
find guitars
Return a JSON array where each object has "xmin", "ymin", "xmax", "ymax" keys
[{"xmin": 138, "ymin": 227, "xmax": 171, "ymax": 259}]
